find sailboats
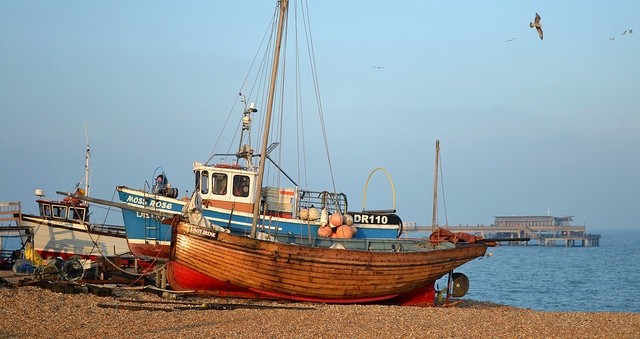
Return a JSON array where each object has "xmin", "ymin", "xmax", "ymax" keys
[
  {"xmin": 166, "ymin": 0, "xmax": 530, "ymax": 304},
  {"xmin": 12, "ymin": 120, "xmax": 158, "ymax": 272}
]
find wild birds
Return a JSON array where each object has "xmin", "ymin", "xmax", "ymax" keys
[
  {"xmin": 621, "ymin": 31, "xmax": 626, "ymax": 35},
  {"xmin": 529, "ymin": 12, "xmax": 544, "ymax": 40},
  {"xmin": 630, "ymin": 29, "xmax": 633, "ymax": 34},
  {"xmin": 505, "ymin": 37, "xmax": 516, "ymax": 42},
  {"xmin": 610, "ymin": 38, "xmax": 615, "ymax": 40}
]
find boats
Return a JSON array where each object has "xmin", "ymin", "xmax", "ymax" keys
[{"xmin": 116, "ymin": 93, "xmax": 403, "ymax": 264}]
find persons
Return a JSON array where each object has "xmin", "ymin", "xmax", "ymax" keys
[{"xmin": 152, "ymin": 175, "xmax": 167, "ymax": 196}]
